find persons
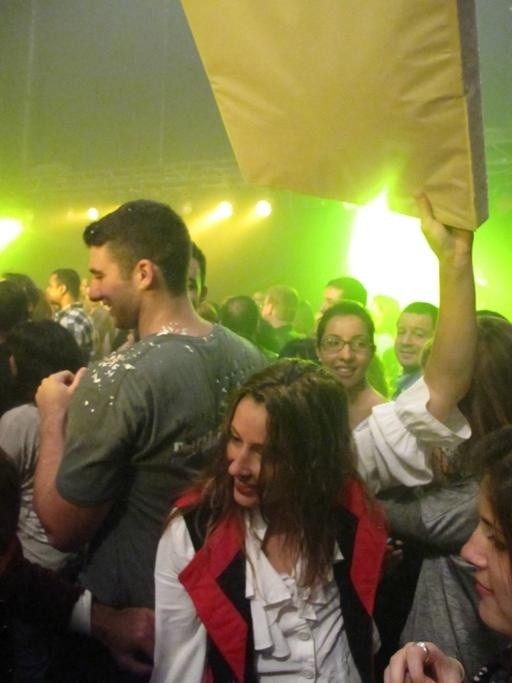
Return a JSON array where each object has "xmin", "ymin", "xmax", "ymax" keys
[
  {"xmin": 151, "ymin": 178, "xmax": 476, "ymax": 683},
  {"xmin": 30, "ymin": 196, "xmax": 271, "ymax": 656}
]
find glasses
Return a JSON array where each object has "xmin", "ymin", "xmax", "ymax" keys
[{"xmin": 320, "ymin": 334, "xmax": 376, "ymax": 353}]
